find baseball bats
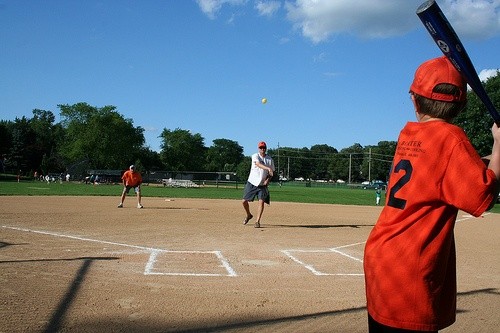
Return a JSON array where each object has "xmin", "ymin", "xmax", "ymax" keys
[{"xmin": 416, "ymin": 0, "xmax": 500, "ymax": 130}]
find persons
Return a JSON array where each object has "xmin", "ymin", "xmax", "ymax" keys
[
  {"xmin": 118, "ymin": 165, "xmax": 143, "ymax": 209},
  {"xmin": 17, "ymin": 169, "xmax": 101, "ymax": 186},
  {"xmin": 242, "ymin": 141, "xmax": 276, "ymax": 228},
  {"xmin": 375, "ymin": 184, "xmax": 382, "ymax": 206},
  {"xmin": 363, "ymin": 54, "xmax": 500, "ymax": 333}
]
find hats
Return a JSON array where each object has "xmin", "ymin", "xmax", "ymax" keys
[
  {"xmin": 130, "ymin": 165, "xmax": 135, "ymax": 169},
  {"xmin": 410, "ymin": 54, "xmax": 467, "ymax": 101},
  {"xmin": 258, "ymin": 142, "xmax": 266, "ymax": 148}
]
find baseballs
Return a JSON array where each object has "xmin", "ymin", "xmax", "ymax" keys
[{"xmin": 262, "ymin": 99, "xmax": 267, "ymax": 103}]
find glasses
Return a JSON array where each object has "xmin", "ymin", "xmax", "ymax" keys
[{"xmin": 259, "ymin": 146, "xmax": 266, "ymax": 149}]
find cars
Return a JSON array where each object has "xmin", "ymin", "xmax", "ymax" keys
[{"xmin": 362, "ymin": 180, "xmax": 386, "ymax": 190}]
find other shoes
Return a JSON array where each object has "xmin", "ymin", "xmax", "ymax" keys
[
  {"xmin": 138, "ymin": 204, "xmax": 143, "ymax": 208},
  {"xmin": 118, "ymin": 204, "xmax": 123, "ymax": 207},
  {"xmin": 255, "ymin": 222, "xmax": 260, "ymax": 228},
  {"xmin": 243, "ymin": 214, "xmax": 253, "ymax": 224}
]
